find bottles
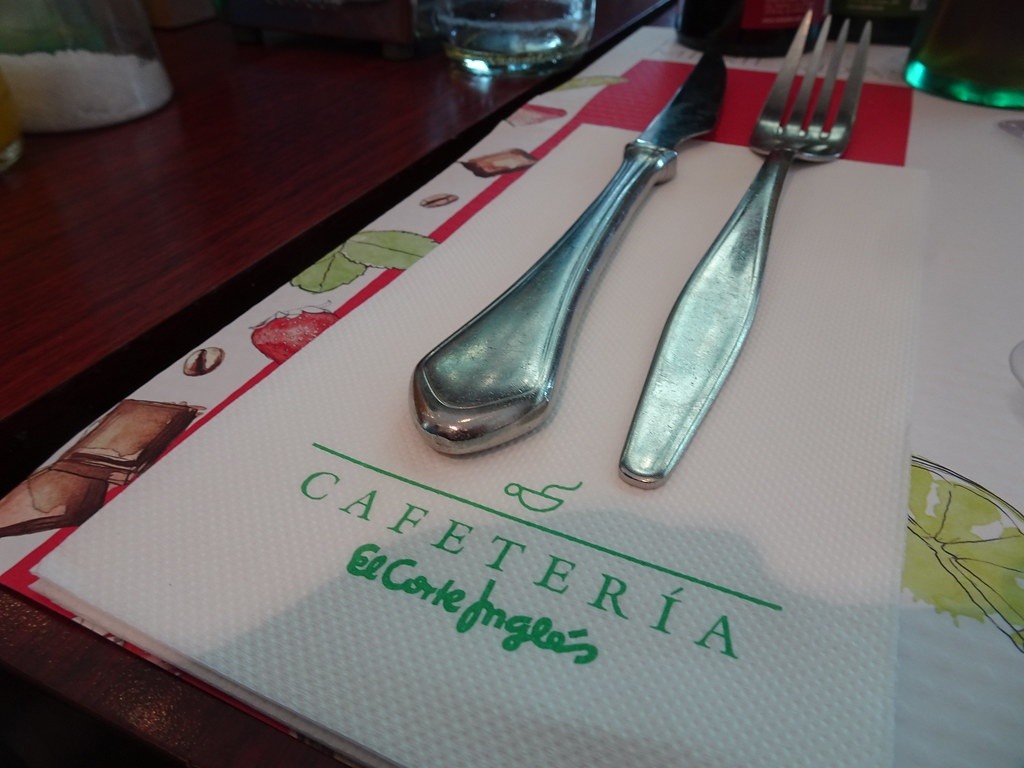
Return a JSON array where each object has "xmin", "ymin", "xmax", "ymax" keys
[
  {"xmin": 2, "ymin": 1, "xmax": 171, "ymax": 129},
  {"xmin": 900, "ymin": 1, "xmax": 1024, "ymax": 108}
]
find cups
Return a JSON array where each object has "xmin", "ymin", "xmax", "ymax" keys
[{"xmin": 420, "ymin": 0, "xmax": 598, "ymax": 78}]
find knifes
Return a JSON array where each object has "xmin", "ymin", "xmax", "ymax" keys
[{"xmin": 410, "ymin": 43, "xmax": 728, "ymax": 456}]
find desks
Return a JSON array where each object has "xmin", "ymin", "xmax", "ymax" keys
[{"xmin": 0, "ymin": 0, "xmax": 1024, "ymax": 768}]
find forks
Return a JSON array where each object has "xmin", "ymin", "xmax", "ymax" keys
[{"xmin": 620, "ymin": 9, "xmax": 872, "ymax": 490}]
